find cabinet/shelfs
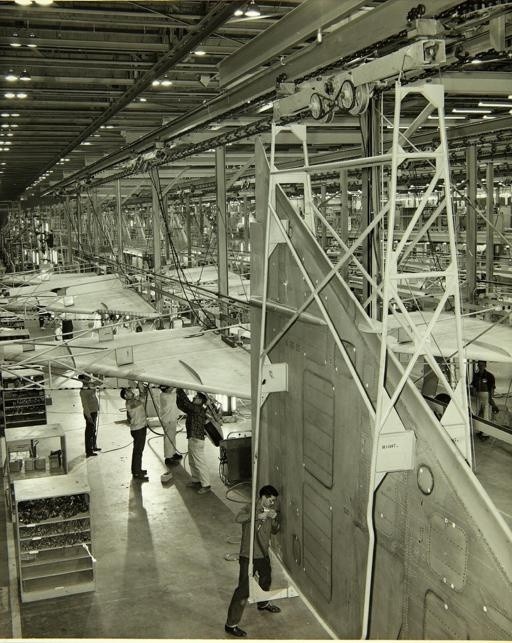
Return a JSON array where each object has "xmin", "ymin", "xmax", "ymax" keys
[{"xmin": 0, "ymin": 367, "xmax": 96, "ymax": 604}]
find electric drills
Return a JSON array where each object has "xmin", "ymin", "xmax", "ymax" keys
[{"xmin": 257, "ymin": 508, "xmax": 278, "ymax": 533}]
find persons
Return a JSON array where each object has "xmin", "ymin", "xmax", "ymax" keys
[
  {"xmin": 78, "ymin": 372, "xmax": 106, "ymax": 458},
  {"xmin": 158, "ymin": 383, "xmax": 183, "ymax": 467},
  {"xmin": 38, "ymin": 306, "xmax": 47, "ymax": 330},
  {"xmin": 120, "ymin": 380, "xmax": 149, "ymax": 483},
  {"xmin": 223, "ymin": 484, "xmax": 283, "ymax": 639},
  {"xmin": 470, "ymin": 360, "xmax": 499, "ymax": 444},
  {"xmin": 175, "ymin": 386, "xmax": 213, "ymax": 496}
]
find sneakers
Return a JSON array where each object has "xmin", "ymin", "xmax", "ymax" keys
[
  {"xmin": 140, "ymin": 470, "xmax": 147, "ymax": 474},
  {"xmin": 198, "ymin": 487, "xmax": 210, "ymax": 494},
  {"xmin": 134, "ymin": 474, "xmax": 148, "ymax": 480},
  {"xmin": 85, "ymin": 447, "xmax": 102, "ymax": 458},
  {"xmin": 185, "ymin": 480, "xmax": 202, "ymax": 489},
  {"xmin": 165, "ymin": 458, "xmax": 179, "ymax": 465},
  {"xmin": 171, "ymin": 453, "xmax": 183, "ymax": 460}
]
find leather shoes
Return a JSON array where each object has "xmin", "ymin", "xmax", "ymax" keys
[
  {"xmin": 258, "ymin": 604, "xmax": 280, "ymax": 613},
  {"xmin": 225, "ymin": 624, "xmax": 247, "ymax": 636}
]
